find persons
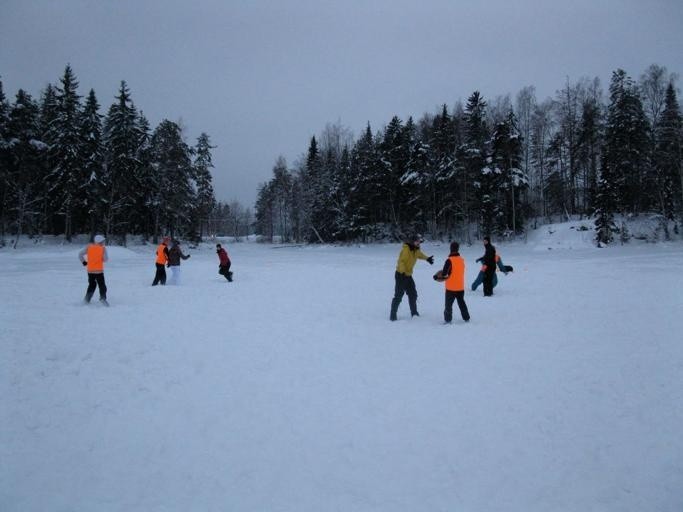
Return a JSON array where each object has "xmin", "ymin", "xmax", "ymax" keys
[
  {"xmin": 215, "ymin": 244, "xmax": 232, "ymax": 282},
  {"xmin": 78, "ymin": 235, "xmax": 109, "ymax": 307},
  {"xmin": 475, "ymin": 237, "xmax": 495, "ymax": 297},
  {"xmin": 388, "ymin": 233, "xmax": 433, "ymax": 320},
  {"xmin": 165, "ymin": 241, "xmax": 190, "ymax": 285},
  {"xmin": 433, "ymin": 242, "xmax": 469, "ymax": 324},
  {"xmin": 151, "ymin": 236, "xmax": 170, "ymax": 285},
  {"xmin": 471, "ymin": 247, "xmax": 512, "ymax": 291}
]
